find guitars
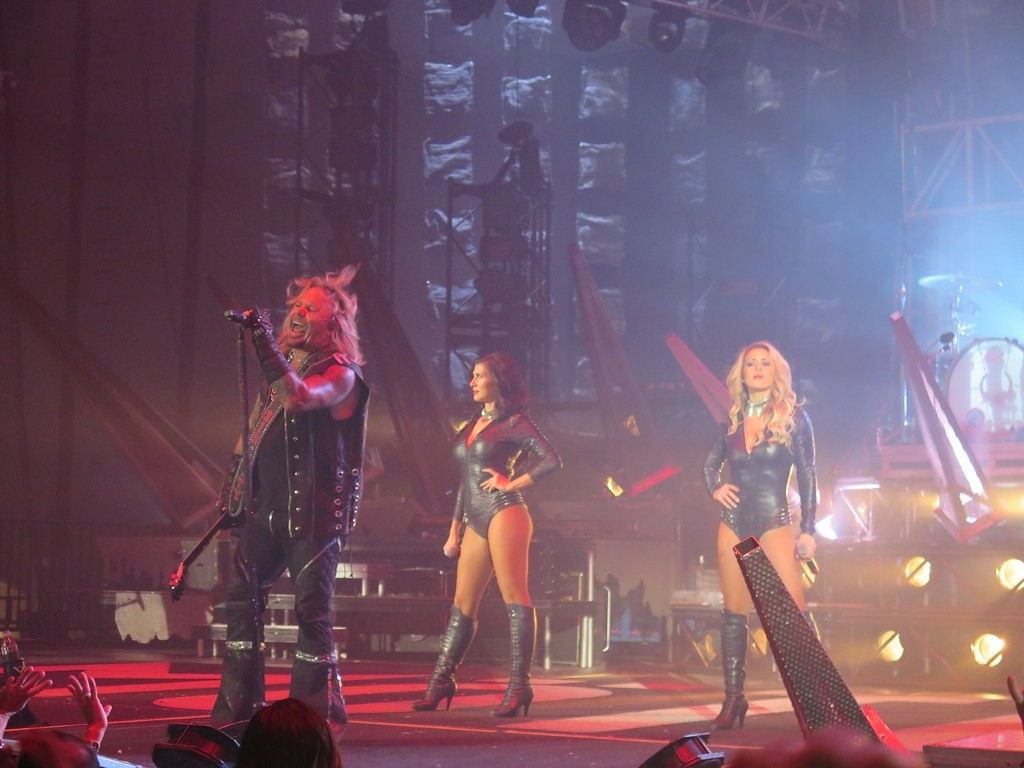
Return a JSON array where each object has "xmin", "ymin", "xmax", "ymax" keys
[{"xmin": 168, "ymin": 510, "xmax": 226, "ymax": 604}]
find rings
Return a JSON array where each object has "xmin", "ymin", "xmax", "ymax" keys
[{"xmin": 83, "ymin": 692, "xmax": 91, "ymax": 696}]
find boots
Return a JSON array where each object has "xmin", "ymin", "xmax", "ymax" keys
[
  {"xmin": 706, "ymin": 612, "xmax": 750, "ymax": 733},
  {"xmin": 409, "ymin": 604, "xmax": 479, "ymax": 713},
  {"xmin": 491, "ymin": 604, "xmax": 538, "ymax": 716},
  {"xmin": 802, "ymin": 611, "xmax": 821, "ymax": 641}
]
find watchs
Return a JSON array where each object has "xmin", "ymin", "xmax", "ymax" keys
[{"xmin": 85, "ymin": 738, "xmax": 101, "ymax": 754}]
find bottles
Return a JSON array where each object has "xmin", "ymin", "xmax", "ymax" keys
[{"xmin": 0, "ymin": 630, "xmax": 21, "ymax": 673}]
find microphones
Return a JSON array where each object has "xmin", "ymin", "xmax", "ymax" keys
[
  {"xmin": 444, "ymin": 540, "xmax": 463, "ymax": 557},
  {"xmin": 224, "ymin": 309, "xmax": 258, "ymax": 328},
  {"xmin": 795, "ymin": 545, "xmax": 819, "ymax": 575}
]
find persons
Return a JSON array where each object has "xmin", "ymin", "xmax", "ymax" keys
[
  {"xmin": 704, "ymin": 339, "xmax": 818, "ymax": 728},
  {"xmin": 0, "ymin": 625, "xmax": 342, "ymax": 768},
  {"xmin": 208, "ymin": 262, "xmax": 368, "ymax": 724},
  {"xmin": 410, "ymin": 350, "xmax": 565, "ymax": 718}
]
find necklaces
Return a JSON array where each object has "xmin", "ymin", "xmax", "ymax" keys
[
  {"xmin": 747, "ymin": 398, "xmax": 772, "ymax": 417},
  {"xmin": 228, "ymin": 354, "xmax": 340, "ymax": 529},
  {"xmin": 478, "ymin": 407, "xmax": 496, "ymax": 420},
  {"xmin": 293, "ymin": 352, "xmax": 307, "ymax": 371}
]
